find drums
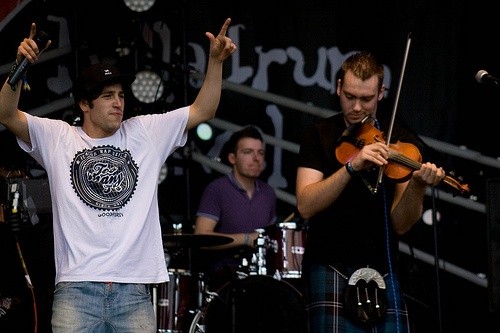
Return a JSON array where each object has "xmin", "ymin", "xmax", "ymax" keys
[
  {"xmin": 262, "ymin": 221, "xmax": 310, "ymax": 280},
  {"xmin": 204, "ymin": 273, "xmax": 304, "ymax": 333},
  {"xmin": 149, "ymin": 268, "xmax": 207, "ymax": 333}
]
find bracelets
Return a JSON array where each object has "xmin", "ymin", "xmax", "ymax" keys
[
  {"xmin": 345, "ymin": 160, "xmax": 356, "ymax": 177},
  {"xmin": 244, "ymin": 232, "xmax": 249, "ymax": 245}
]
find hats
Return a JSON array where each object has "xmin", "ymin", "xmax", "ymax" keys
[{"xmin": 73, "ymin": 63, "xmax": 136, "ymax": 109}]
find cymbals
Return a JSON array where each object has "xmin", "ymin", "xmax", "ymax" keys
[{"xmin": 161, "ymin": 234, "xmax": 235, "ymax": 248}]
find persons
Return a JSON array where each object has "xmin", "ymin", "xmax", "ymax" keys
[
  {"xmin": 0, "ymin": 18, "xmax": 236, "ymax": 333},
  {"xmin": 296, "ymin": 52, "xmax": 446, "ymax": 333},
  {"xmin": 194, "ymin": 126, "xmax": 277, "ymax": 284}
]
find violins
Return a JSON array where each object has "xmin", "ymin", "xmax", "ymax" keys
[{"xmin": 334, "ymin": 121, "xmax": 471, "ymax": 198}]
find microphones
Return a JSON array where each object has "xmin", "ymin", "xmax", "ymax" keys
[
  {"xmin": 475, "ymin": 69, "xmax": 500, "ymax": 93},
  {"xmin": 8, "ymin": 31, "xmax": 49, "ymax": 87}
]
